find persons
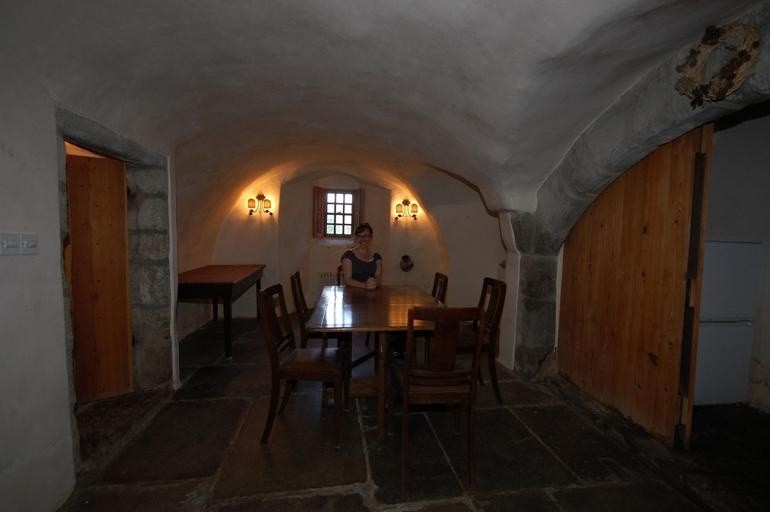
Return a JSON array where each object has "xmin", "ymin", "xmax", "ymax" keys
[{"xmin": 341, "ymin": 222, "xmax": 405, "ymax": 378}]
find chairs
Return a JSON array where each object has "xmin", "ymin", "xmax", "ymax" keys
[
  {"xmin": 257, "ymin": 270, "xmax": 353, "ymax": 456},
  {"xmin": 400, "ymin": 272, "xmax": 508, "ymax": 494}
]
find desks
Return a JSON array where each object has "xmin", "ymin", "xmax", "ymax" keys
[
  {"xmin": 306, "ymin": 283, "xmax": 474, "ymax": 453},
  {"xmin": 177, "ymin": 263, "xmax": 266, "ymax": 362}
]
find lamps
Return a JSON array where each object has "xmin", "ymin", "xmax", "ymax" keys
[
  {"xmin": 247, "ymin": 193, "xmax": 273, "ymax": 216},
  {"xmin": 395, "ymin": 198, "xmax": 418, "ymax": 221}
]
